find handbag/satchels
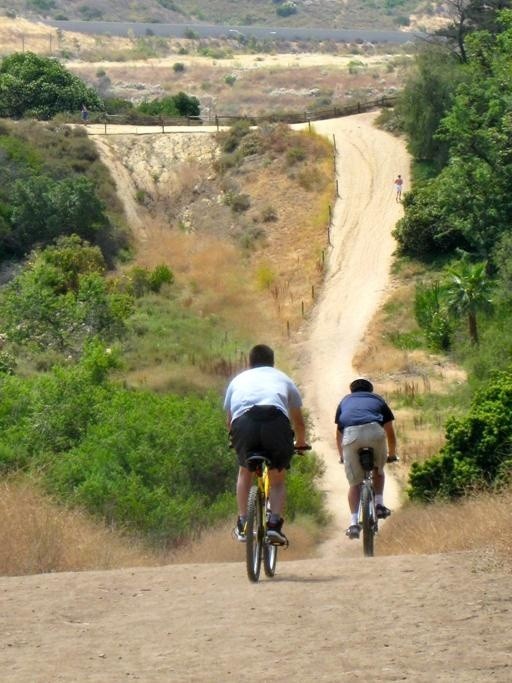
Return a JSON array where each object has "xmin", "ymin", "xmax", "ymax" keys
[{"xmin": 249, "ymin": 406, "xmax": 280, "ymax": 419}]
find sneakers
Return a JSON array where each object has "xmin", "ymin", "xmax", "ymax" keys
[
  {"xmin": 376, "ymin": 502, "xmax": 392, "ymax": 519},
  {"xmin": 346, "ymin": 523, "xmax": 359, "ymax": 539}
]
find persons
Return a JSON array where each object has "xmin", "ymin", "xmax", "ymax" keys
[
  {"xmin": 224, "ymin": 343, "xmax": 306, "ymax": 544},
  {"xmin": 393, "ymin": 174, "xmax": 404, "ymax": 202},
  {"xmin": 334, "ymin": 378, "xmax": 396, "ymax": 537}
]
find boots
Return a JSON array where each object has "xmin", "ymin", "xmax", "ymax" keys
[
  {"xmin": 231, "ymin": 518, "xmax": 247, "ymax": 539},
  {"xmin": 266, "ymin": 514, "xmax": 286, "ymax": 542}
]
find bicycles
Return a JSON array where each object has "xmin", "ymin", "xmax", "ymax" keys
[{"xmin": 228, "ymin": 442, "xmax": 397, "ymax": 583}]
